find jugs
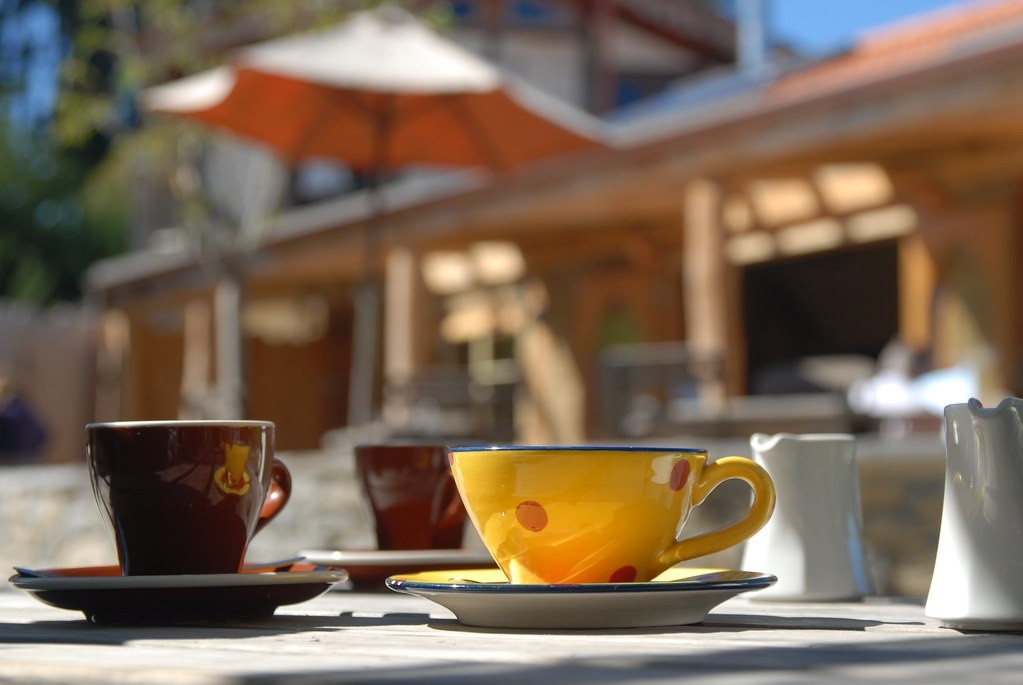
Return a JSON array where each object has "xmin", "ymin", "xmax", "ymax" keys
[
  {"xmin": 740, "ymin": 433, "xmax": 868, "ymax": 603},
  {"xmin": 921, "ymin": 397, "xmax": 1023, "ymax": 630}
]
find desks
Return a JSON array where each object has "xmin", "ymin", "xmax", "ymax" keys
[{"xmin": 2, "ymin": 579, "xmax": 1020, "ymax": 685}]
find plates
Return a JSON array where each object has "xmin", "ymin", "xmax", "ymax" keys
[
  {"xmin": 297, "ymin": 551, "xmax": 500, "ymax": 592},
  {"xmin": 10, "ymin": 566, "xmax": 348, "ymax": 623},
  {"xmin": 385, "ymin": 567, "xmax": 777, "ymax": 630}
]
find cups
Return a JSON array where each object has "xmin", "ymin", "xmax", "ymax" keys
[
  {"xmin": 86, "ymin": 419, "xmax": 291, "ymax": 575},
  {"xmin": 446, "ymin": 446, "xmax": 776, "ymax": 586},
  {"xmin": 354, "ymin": 444, "xmax": 468, "ymax": 550}
]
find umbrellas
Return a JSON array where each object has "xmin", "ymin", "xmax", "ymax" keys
[{"xmin": 131, "ymin": 1, "xmax": 627, "ymax": 424}]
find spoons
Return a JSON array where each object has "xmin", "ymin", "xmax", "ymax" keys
[
  {"xmin": 14, "ymin": 555, "xmax": 303, "ymax": 581},
  {"xmin": 450, "ymin": 572, "xmax": 738, "ymax": 595}
]
